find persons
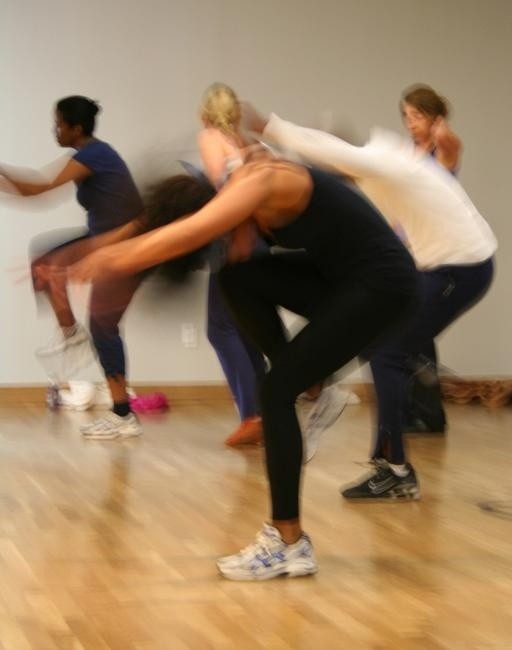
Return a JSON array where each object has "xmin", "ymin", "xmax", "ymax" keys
[
  {"xmin": 234, "ymin": 97, "xmax": 499, "ymax": 508},
  {"xmin": 0, "ymin": 94, "xmax": 151, "ymax": 443},
  {"xmin": 381, "ymin": 81, "xmax": 462, "ymax": 435},
  {"xmin": 195, "ymin": 78, "xmax": 279, "ymax": 452},
  {"xmin": 6, "ymin": 151, "xmax": 429, "ymax": 585}
]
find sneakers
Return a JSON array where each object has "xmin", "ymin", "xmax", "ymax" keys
[
  {"xmin": 78, "ymin": 408, "xmax": 144, "ymax": 440},
  {"xmin": 214, "ymin": 521, "xmax": 318, "ymax": 582},
  {"xmin": 398, "ymin": 415, "xmax": 447, "ymax": 433},
  {"xmin": 342, "ymin": 460, "xmax": 425, "ymax": 500},
  {"xmin": 291, "ymin": 386, "xmax": 350, "ymax": 464},
  {"xmin": 222, "ymin": 414, "xmax": 264, "ymax": 447},
  {"xmin": 48, "ymin": 384, "xmax": 169, "ymax": 411},
  {"xmin": 34, "ymin": 321, "xmax": 89, "ymax": 359}
]
